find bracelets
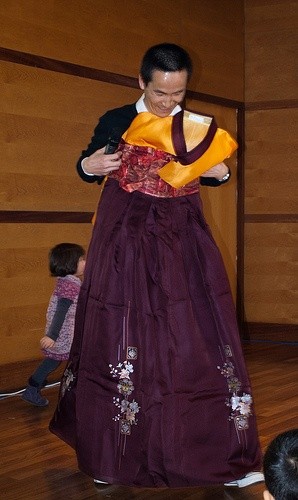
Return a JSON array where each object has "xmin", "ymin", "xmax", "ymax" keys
[{"xmin": 219, "ymin": 173, "xmax": 230, "ymax": 181}]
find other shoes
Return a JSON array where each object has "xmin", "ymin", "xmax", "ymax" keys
[{"xmin": 224, "ymin": 471, "xmax": 264, "ymax": 488}]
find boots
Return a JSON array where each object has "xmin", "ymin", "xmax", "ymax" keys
[{"xmin": 20, "ymin": 384, "xmax": 49, "ymax": 406}]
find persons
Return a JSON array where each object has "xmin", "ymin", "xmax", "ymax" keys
[
  {"xmin": 261, "ymin": 426, "xmax": 298, "ymax": 500},
  {"xmin": 47, "ymin": 44, "xmax": 269, "ymax": 500},
  {"xmin": 19, "ymin": 243, "xmax": 85, "ymax": 406}
]
